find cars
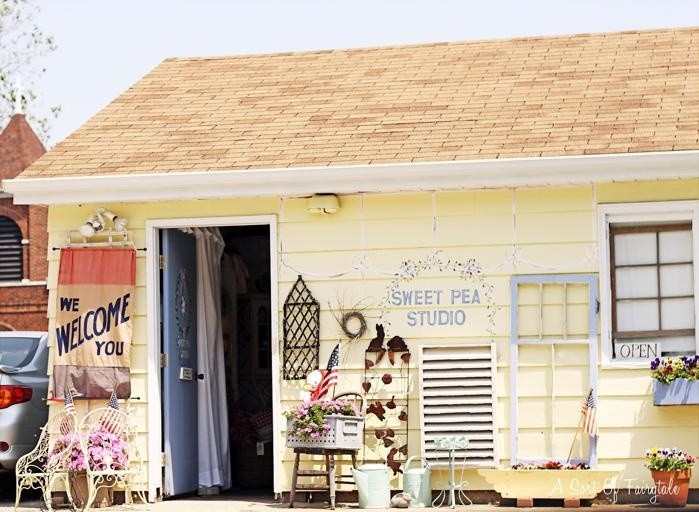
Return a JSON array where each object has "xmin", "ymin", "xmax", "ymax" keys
[{"xmin": 1, "ymin": 329, "xmax": 49, "ymax": 476}]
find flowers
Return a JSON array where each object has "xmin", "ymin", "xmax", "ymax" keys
[
  {"xmin": 511, "ymin": 460, "xmax": 599, "ymax": 470},
  {"xmin": 281, "ymin": 399, "xmax": 363, "ymax": 437},
  {"xmin": 43, "ymin": 425, "xmax": 132, "ymax": 472},
  {"xmin": 642, "ymin": 446, "xmax": 696, "ymax": 471},
  {"xmin": 650, "ymin": 353, "xmax": 699, "ymax": 382}
]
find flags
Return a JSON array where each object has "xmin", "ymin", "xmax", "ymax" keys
[
  {"xmin": 579, "ymin": 389, "xmax": 599, "ymax": 471},
  {"xmin": 60, "ymin": 390, "xmax": 75, "ymax": 436},
  {"xmin": 100, "ymin": 390, "xmax": 122, "ymax": 442},
  {"xmin": 307, "ymin": 342, "xmax": 340, "ymax": 401}
]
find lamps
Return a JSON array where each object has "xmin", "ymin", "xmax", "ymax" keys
[
  {"xmin": 80, "ymin": 207, "xmax": 131, "ymax": 239},
  {"xmin": 306, "ymin": 192, "xmax": 343, "ymax": 217}
]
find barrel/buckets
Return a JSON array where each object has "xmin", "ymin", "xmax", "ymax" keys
[
  {"xmin": 351, "ymin": 464, "xmax": 391, "ymax": 509},
  {"xmin": 403, "ymin": 456, "xmax": 432, "ymax": 507}
]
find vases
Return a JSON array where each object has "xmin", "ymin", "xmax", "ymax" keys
[
  {"xmin": 651, "ymin": 379, "xmax": 699, "ymax": 405},
  {"xmin": 69, "ymin": 474, "xmax": 114, "ymax": 507},
  {"xmin": 650, "ymin": 466, "xmax": 693, "ymax": 506}
]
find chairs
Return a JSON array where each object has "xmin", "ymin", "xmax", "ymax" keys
[
  {"xmin": 288, "ymin": 391, "xmax": 368, "ymax": 511},
  {"xmin": 79, "ymin": 407, "xmax": 149, "ymax": 511},
  {"xmin": 12, "ymin": 409, "xmax": 77, "ymax": 512}
]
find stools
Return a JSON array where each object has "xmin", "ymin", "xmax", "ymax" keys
[{"xmin": 431, "ymin": 435, "xmax": 472, "ymax": 510}]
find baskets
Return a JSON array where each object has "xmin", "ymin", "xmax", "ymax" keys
[{"xmin": 285, "ymin": 413, "xmax": 364, "ymax": 451}]
